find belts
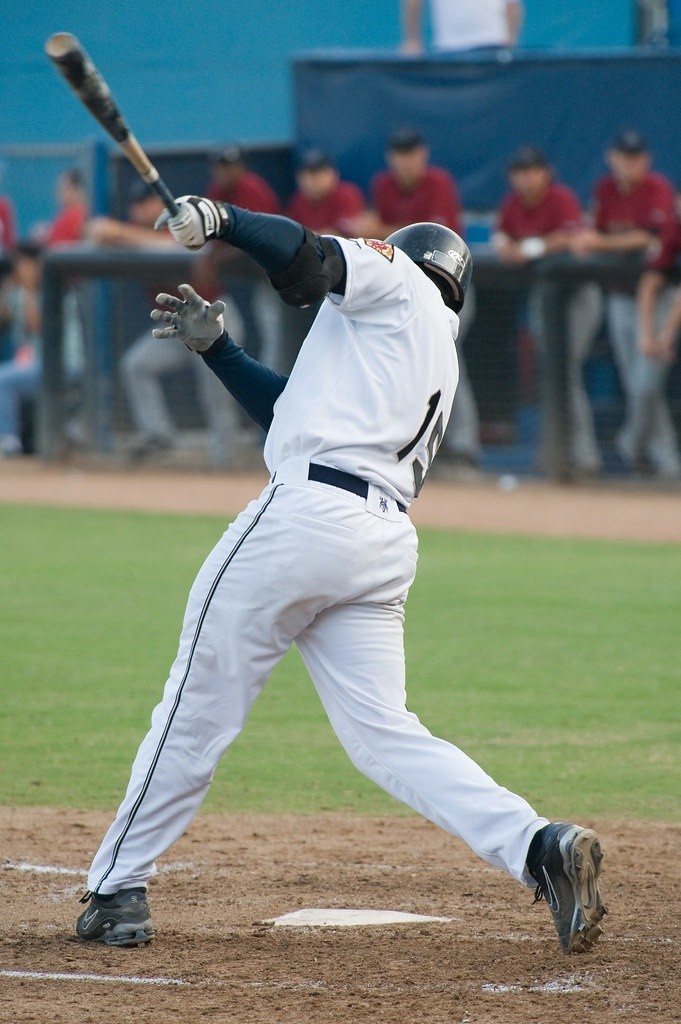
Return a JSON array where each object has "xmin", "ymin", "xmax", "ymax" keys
[{"xmin": 272, "ymin": 463, "xmax": 408, "ymax": 514}]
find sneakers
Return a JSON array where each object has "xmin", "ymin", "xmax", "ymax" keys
[
  {"xmin": 529, "ymin": 820, "xmax": 609, "ymax": 956},
  {"xmin": 76, "ymin": 886, "xmax": 155, "ymax": 947}
]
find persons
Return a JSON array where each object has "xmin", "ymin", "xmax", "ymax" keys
[
  {"xmin": 0, "ymin": 168, "xmax": 99, "ymax": 454},
  {"xmin": 372, "ymin": 131, "xmax": 482, "ymax": 457},
  {"xmin": 203, "ymin": 144, "xmax": 287, "ymax": 373},
  {"xmin": 577, "ymin": 128, "xmax": 680, "ymax": 480},
  {"xmin": 77, "ymin": 190, "xmax": 609, "ymax": 954},
  {"xmin": 490, "ymin": 144, "xmax": 609, "ymax": 471},
  {"xmin": 86, "ymin": 179, "xmax": 247, "ymax": 456},
  {"xmin": 290, "ymin": 151, "xmax": 364, "ymax": 235},
  {"xmin": 399, "ymin": 0, "xmax": 525, "ymax": 58}
]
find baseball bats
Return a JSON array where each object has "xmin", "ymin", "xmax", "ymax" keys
[{"xmin": 45, "ymin": 32, "xmax": 182, "ymax": 221}]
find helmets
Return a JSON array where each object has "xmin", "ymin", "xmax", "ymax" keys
[{"xmin": 382, "ymin": 222, "xmax": 473, "ymax": 315}]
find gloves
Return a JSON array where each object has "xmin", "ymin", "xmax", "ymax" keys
[
  {"xmin": 154, "ymin": 195, "xmax": 229, "ymax": 252},
  {"xmin": 150, "ymin": 283, "xmax": 228, "ymax": 356}
]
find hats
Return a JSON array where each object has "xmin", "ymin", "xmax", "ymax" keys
[
  {"xmin": 385, "ymin": 128, "xmax": 428, "ymax": 148},
  {"xmin": 610, "ymin": 128, "xmax": 647, "ymax": 153}
]
